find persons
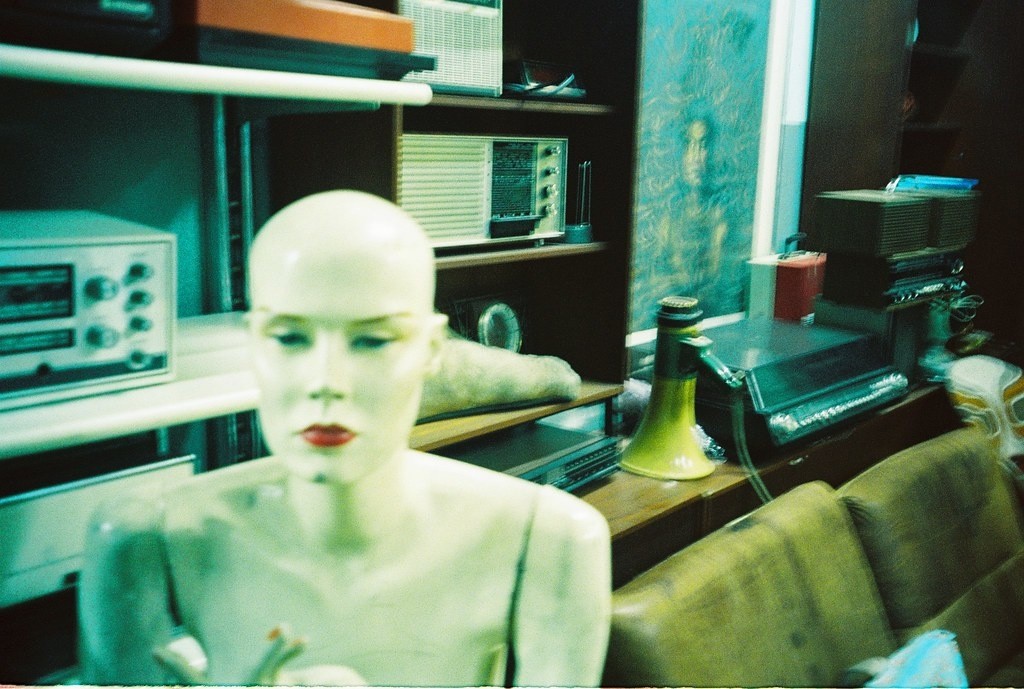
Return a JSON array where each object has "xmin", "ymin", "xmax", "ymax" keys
[{"xmin": 79, "ymin": 189, "xmax": 612, "ymax": 687}]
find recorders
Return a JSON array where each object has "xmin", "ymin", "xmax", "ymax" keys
[{"xmin": 398, "ymin": 132, "xmax": 569, "ymax": 248}]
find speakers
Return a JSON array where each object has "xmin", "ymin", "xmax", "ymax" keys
[{"xmin": 811, "ymin": 185, "xmax": 984, "ymax": 257}]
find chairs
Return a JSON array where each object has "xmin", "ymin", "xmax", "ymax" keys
[
  {"xmin": 600, "ymin": 476, "xmax": 897, "ymax": 687},
  {"xmin": 833, "ymin": 422, "xmax": 1024, "ymax": 689}
]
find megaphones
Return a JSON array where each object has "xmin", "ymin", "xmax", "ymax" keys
[{"xmin": 617, "ymin": 296, "xmax": 742, "ymax": 481}]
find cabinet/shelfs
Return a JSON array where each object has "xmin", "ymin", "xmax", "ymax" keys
[
  {"xmin": 267, "ymin": 0, "xmax": 707, "ymax": 591},
  {"xmin": 622, "ymin": 345, "xmax": 940, "ymax": 536},
  {"xmin": 800, "ymin": 0, "xmax": 1023, "ymax": 350},
  {"xmin": 0, "ymin": 38, "xmax": 438, "ymax": 687}
]
